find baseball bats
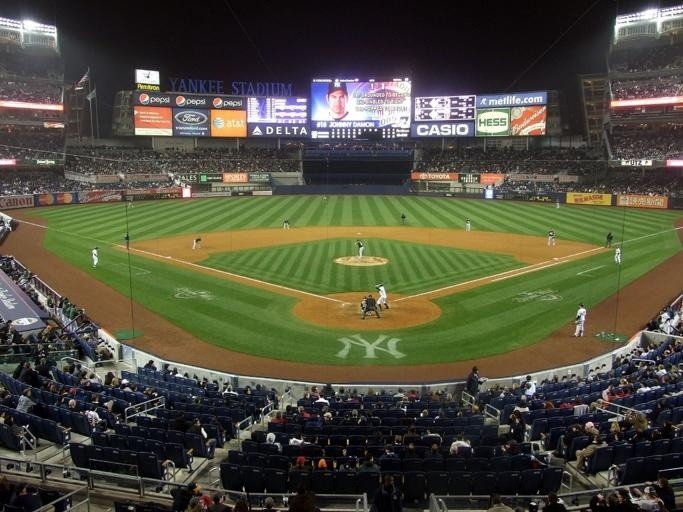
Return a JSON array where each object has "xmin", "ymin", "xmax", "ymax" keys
[{"xmin": 377, "ymin": 281, "xmax": 387, "ymax": 287}]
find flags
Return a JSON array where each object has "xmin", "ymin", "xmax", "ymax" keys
[{"xmin": 75, "ymin": 72, "xmax": 96, "ymax": 101}]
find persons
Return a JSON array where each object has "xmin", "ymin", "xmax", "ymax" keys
[
  {"xmin": 191, "ymin": 236, "xmax": 202, "ymax": 251},
  {"xmin": 375, "ymin": 284, "xmax": 389, "ymax": 311},
  {"xmin": 282, "ymin": 216, "xmax": 290, "ymax": 229},
  {"xmin": 603, "ymin": 232, "xmax": 613, "ymax": 248},
  {"xmin": 464, "ymin": 216, "xmax": 470, "ymax": 233},
  {"xmin": 605, "ymin": 30, "xmax": 683, "ymax": 201},
  {"xmin": 0, "ymin": 303, "xmax": 683, "ymax": 510},
  {"xmin": 400, "ymin": 213, "xmax": 406, "ymax": 224},
  {"xmin": 556, "ymin": 199, "xmax": 559, "ymax": 209},
  {"xmin": 61, "ymin": 140, "xmax": 302, "ymax": 209},
  {"xmin": 325, "ymin": 81, "xmax": 351, "ymax": 120},
  {"xmin": 0, "ymin": 214, "xmax": 69, "ymax": 328},
  {"xmin": 415, "ymin": 141, "xmax": 616, "ymax": 201},
  {"xmin": 360, "ymin": 294, "xmax": 380, "ymax": 320},
  {"xmin": 90, "ymin": 246, "xmax": 99, "ymax": 270},
  {"xmin": 124, "ymin": 229, "xmax": 130, "ymax": 251},
  {"xmin": 352, "ymin": 239, "xmax": 364, "ymax": 260},
  {"xmin": 2, "ymin": 53, "xmax": 68, "ymax": 197},
  {"xmin": 544, "ymin": 227, "xmax": 555, "ymax": 248},
  {"xmin": 614, "ymin": 246, "xmax": 621, "ymax": 265},
  {"xmin": 359, "ymin": 296, "xmax": 379, "ymax": 316}
]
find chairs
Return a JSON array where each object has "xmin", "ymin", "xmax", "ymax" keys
[{"xmin": 0, "ymin": 331, "xmax": 683, "ymax": 512}]
